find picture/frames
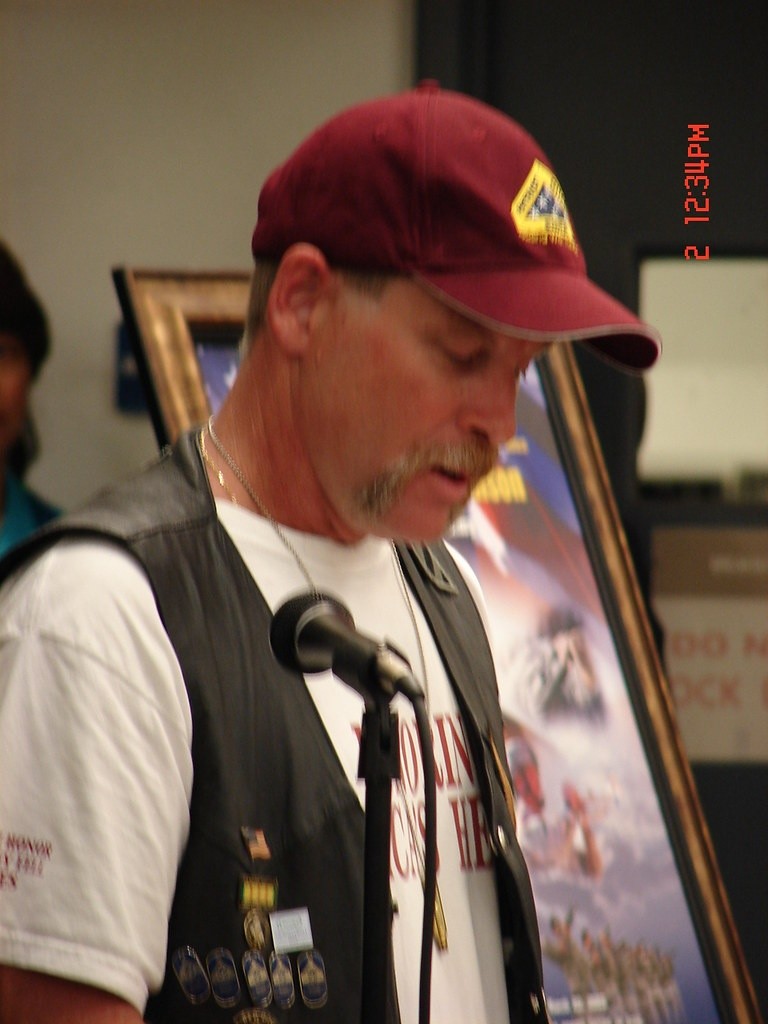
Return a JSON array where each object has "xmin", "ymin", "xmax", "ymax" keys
[{"xmin": 111, "ymin": 262, "xmax": 761, "ymax": 1024}]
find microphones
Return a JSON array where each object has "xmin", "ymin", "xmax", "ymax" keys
[{"xmin": 270, "ymin": 592, "xmax": 425, "ymax": 700}]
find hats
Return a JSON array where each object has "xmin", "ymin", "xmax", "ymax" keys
[
  {"xmin": 0, "ymin": 242, "xmax": 51, "ymax": 377},
  {"xmin": 250, "ymin": 76, "xmax": 662, "ymax": 373}
]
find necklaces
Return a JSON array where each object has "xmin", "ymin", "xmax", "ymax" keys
[
  {"xmin": 204, "ymin": 421, "xmax": 451, "ymax": 951},
  {"xmin": 196, "ymin": 419, "xmax": 238, "ymax": 504}
]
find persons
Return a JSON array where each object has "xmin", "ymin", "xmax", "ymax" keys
[
  {"xmin": 0, "ymin": 76, "xmax": 662, "ymax": 1024},
  {"xmin": 542, "ymin": 897, "xmax": 689, "ymax": 1024},
  {"xmin": 0, "ymin": 242, "xmax": 67, "ymax": 569}
]
